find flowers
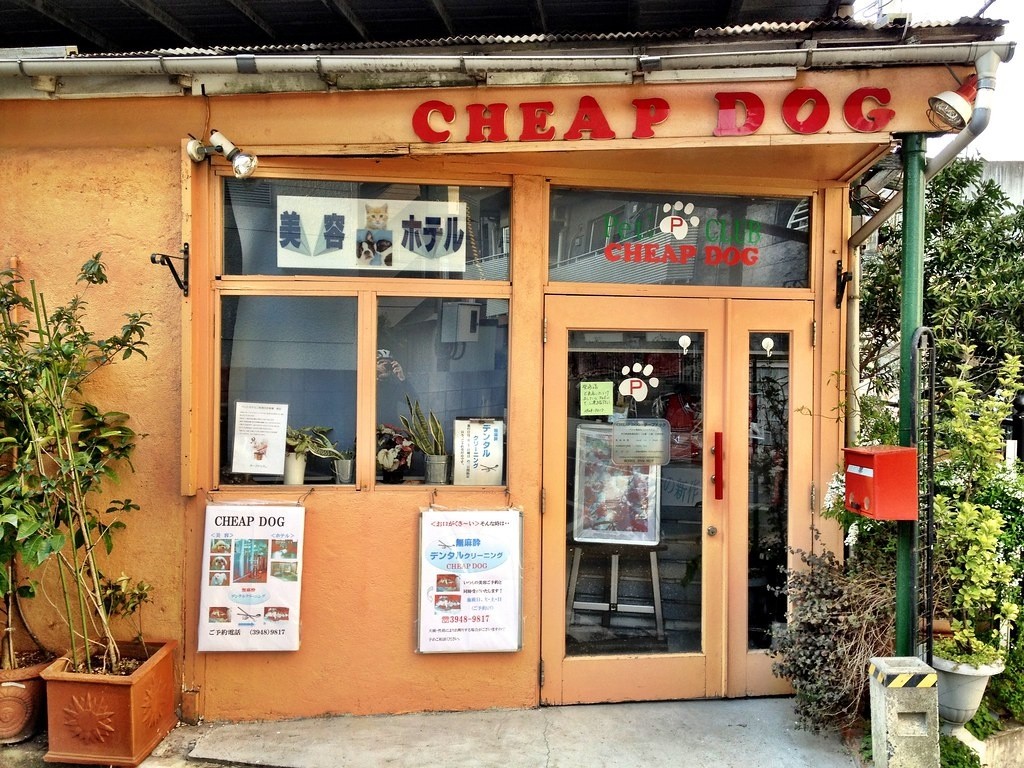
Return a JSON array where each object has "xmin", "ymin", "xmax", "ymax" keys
[{"xmin": 375, "ymin": 423, "xmax": 415, "ymax": 471}]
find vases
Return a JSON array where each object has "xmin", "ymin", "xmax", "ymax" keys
[{"xmin": 382, "ymin": 467, "xmax": 400, "ymax": 484}]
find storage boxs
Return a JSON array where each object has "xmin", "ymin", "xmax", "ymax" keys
[{"xmin": 451, "ymin": 415, "xmax": 506, "ymax": 486}]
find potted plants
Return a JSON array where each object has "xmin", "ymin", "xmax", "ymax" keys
[
  {"xmin": 399, "ymin": 394, "xmax": 447, "ymax": 484},
  {"xmin": 0, "ymin": 271, "xmax": 178, "ymax": 767},
  {"xmin": 310, "ymin": 424, "xmax": 356, "ymax": 484},
  {"xmin": 916, "ymin": 493, "xmax": 1015, "ymax": 724},
  {"xmin": 284, "ymin": 426, "xmax": 314, "ymax": 485}
]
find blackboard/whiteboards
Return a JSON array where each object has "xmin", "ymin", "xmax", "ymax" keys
[{"xmin": 573, "ymin": 421, "xmax": 662, "ymax": 551}]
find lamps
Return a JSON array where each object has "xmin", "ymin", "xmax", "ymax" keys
[{"xmin": 187, "ymin": 129, "xmax": 258, "ymax": 180}]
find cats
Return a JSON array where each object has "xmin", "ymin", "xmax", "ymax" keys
[{"xmin": 364, "ymin": 202, "xmax": 388, "ymax": 230}]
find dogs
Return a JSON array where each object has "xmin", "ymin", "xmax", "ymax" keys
[{"xmin": 356, "ymin": 230, "xmax": 392, "ymax": 266}]
